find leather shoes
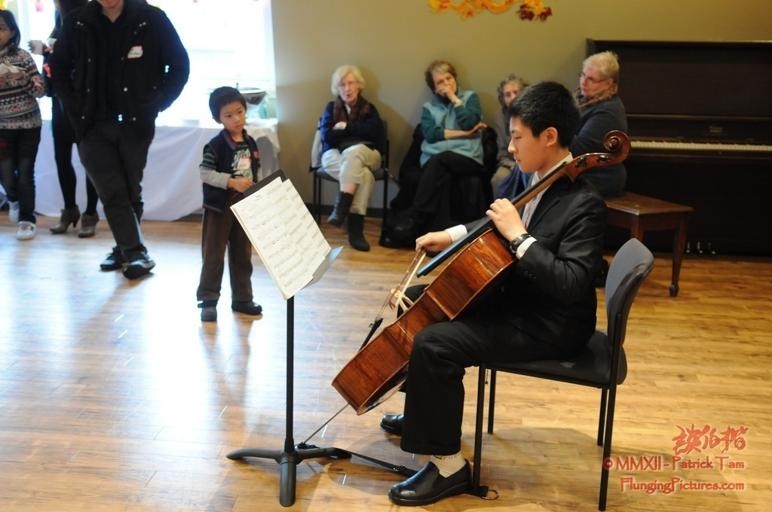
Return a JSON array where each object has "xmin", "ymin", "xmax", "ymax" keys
[
  {"xmin": 387, "ymin": 458, "xmax": 472, "ymax": 506},
  {"xmin": 380, "ymin": 219, "xmax": 413, "ymax": 249},
  {"xmin": 381, "ymin": 414, "xmax": 404, "ymax": 436}
]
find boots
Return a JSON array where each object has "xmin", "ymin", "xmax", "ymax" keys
[
  {"xmin": 348, "ymin": 213, "xmax": 370, "ymax": 251},
  {"xmin": 328, "ymin": 192, "xmax": 354, "ymax": 228}
]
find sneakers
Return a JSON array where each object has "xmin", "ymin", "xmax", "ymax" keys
[
  {"xmin": 17, "ymin": 220, "xmax": 37, "ymax": 240},
  {"xmin": 198, "ymin": 300, "xmax": 217, "ymax": 321},
  {"xmin": 232, "ymin": 300, "xmax": 262, "ymax": 315},
  {"xmin": 8, "ymin": 201, "xmax": 19, "ymax": 222},
  {"xmin": 99, "ymin": 246, "xmax": 122, "ymax": 270},
  {"xmin": 122, "ymin": 244, "xmax": 155, "ymax": 279}
]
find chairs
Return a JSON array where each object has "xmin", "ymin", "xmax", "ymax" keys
[
  {"xmin": 313, "ymin": 117, "xmax": 390, "ymax": 229},
  {"xmin": 473, "ymin": 237, "xmax": 654, "ymax": 512}
]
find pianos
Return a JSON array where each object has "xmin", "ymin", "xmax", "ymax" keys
[{"xmin": 584, "ymin": 39, "xmax": 771, "ymax": 261}]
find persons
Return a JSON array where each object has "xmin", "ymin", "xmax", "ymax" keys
[
  {"xmin": 416, "ymin": 62, "xmax": 492, "ymax": 225},
  {"xmin": 52, "ymin": 0, "xmax": 190, "ymax": 279},
  {"xmin": 29, "ymin": 0, "xmax": 99, "ymax": 237},
  {"xmin": 488, "ymin": 76, "xmax": 530, "ymax": 192},
  {"xmin": 319, "ymin": 66, "xmax": 383, "ymax": 252},
  {"xmin": 495, "ymin": 52, "xmax": 630, "ymax": 213},
  {"xmin": 196, "ymin": 86, "xmax": 263, "ymax": 322},
  {"xmin": 381, "ymin": 81, "xmax": 609, "ymax": 507},
  {"xmin": 0, "ymin": 8, "xmax": 45, "ymax": 241}
]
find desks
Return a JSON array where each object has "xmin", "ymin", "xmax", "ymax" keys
[{"xmin": 34, "ymin": 104, "xmax": 281, "ymax": 222}]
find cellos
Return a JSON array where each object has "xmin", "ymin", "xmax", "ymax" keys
[{"xmin": 330, "ymin": 130, "xmax": 631, "ymax": 416}]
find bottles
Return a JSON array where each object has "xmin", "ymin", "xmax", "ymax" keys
[{"xmin": 258, "ymin": 99, "xmax": 269, "ymax": 119}]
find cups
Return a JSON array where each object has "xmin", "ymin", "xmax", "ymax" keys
[{"xmin": 31, "ymin": 39, "xmax": 43, "ymax": 54}]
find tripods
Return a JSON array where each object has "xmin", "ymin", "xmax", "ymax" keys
[{"xmin": 227, "ymin": 295, "xmax": 353, "ymax": 508}]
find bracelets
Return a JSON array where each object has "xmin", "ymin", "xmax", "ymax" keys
[{"xmin": 511, "ymin": 228, "xmax": 530, "ymax": 252}]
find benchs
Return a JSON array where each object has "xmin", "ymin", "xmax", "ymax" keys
[{"xmin": 603, "ymin": 190, "xmax": 693, "ymax": 297}]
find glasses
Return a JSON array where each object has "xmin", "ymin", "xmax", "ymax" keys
[{"xmin": 578, "ymin": 72, "xmax": 608, "ymax": 83}]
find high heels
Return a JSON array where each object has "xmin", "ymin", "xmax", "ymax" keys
[
  {"xmin": 49, "ymin": 204, "xmax": 80, "ymax": 234},
  {"xmin": 79, "ymin": 212, "xmax": 99, "ymax": 238}
]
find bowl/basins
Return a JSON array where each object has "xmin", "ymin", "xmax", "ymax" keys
[{"xmin": 235, "ymin": 88, "xmax": 268, "ymax": 113}]
